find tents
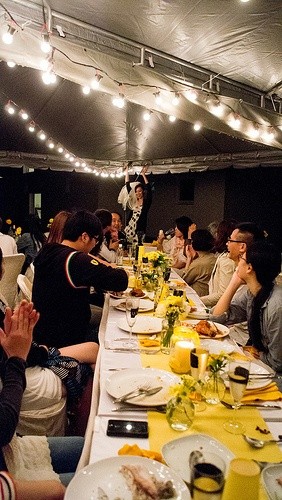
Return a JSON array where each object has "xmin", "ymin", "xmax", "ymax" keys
[{"xmin": 0, "ymin": 0, "xmax": 282, "ymax": 178}]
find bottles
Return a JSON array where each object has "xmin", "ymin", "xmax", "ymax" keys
[{"xmin": 116, "ymin": 244, "xmax": 123, "ymax": 265}]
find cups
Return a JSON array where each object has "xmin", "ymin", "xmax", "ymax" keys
[
  {"xmin": 190, "ymin": 347, "xmax": 209, "ymax": 383},
  {"xmin": 220, "ymin": 457, "xmax": 261, "ymax": 500},
  {"xmin": 188, "ymin": 449, "xmax": 226, "ymax": 500},
  {"xmin": 133, "ymin": 265, "xmax": 141, "ymax": 280}
]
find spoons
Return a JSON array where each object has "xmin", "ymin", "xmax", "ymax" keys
[
  {"xmin": 116, "ymin": 386, "xmax": 163, "ymax": 402},
  {"xmin": 241, "ymin": 434, "xmax": 282, "ymax": 448}
]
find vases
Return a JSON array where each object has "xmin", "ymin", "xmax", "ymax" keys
[
  {"xmin": 165, "ymin": 396, "xmax": 196, "ymax": 431},
  {"xmin": 201, "ymin": 374, "xmax": 226, "ymax": 405},
  {"xmin": 160, "ymin": 323, "xmax": 182, "ymax": 354}
]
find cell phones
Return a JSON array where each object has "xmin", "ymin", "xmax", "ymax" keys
[{"xmin": 107, "ymin": 419, "xmax": 150, "ymax": 439}]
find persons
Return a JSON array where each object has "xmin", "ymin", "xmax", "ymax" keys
[
  {"xmin": 31, "ymin": 209, "xmax": 128, "ymax": 349},
  {"xmin": 226, "ymin": 222, "xmax": 282, "ymax": 286},
  {"xmin": 200, "ymin": 220, "xmax": 236, "ymax": 308},
  {"xmin": 45, "ymin": 209, "xmax": 127, "ymax": 308},
  {"xmin": 157, "ymin": 215, "xmax": 220, "ymax": 297},
  {"xmin": 0, "ymin": 213, "xmax": 99, "ymax": 437},
  {"xmin": 209, "ymin": 234, "xmax": 282, "ymax": 376},
  {"xmin": 0, "ymin": 299, "xmax": 85, "ymax": 487},
  {"xmin": 123, "ymin": 161, "xmax": 153, "ymax": 243},
  {"xmin": 0, "ymin": 471, "xmax": 66, "ymax": 500}
]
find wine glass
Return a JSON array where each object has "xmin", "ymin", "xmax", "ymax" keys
[
  {"xmin": 127, "ymin": 245, "xmax": 134, "ymax": 267},
  {"xmin": 125, "ymin": 296, "xmax": 140, "ymax": 347},
  {"xmin": 223, "ymin": 359, "xmax": 251, "ymax": 435}
]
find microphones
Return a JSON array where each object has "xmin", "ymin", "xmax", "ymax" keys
[{"xmin": 123, "ymin": 161, "xmax": 133, "ymax": 172}]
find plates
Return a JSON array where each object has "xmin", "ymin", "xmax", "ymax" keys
[
  {"xmin": 108, "ymin": 287, "xmax": 147, "ymax": 298},
  {"xmin": 63, "ymin": 455, "xmax": 192, "ymax": 500},
  {"xmin": 183, "ymin": 320, "xmax": 230, "ymax": 339},
  {"xmin": 162, "ymin": 434, "xmax": 235, "ymax": 484},
  {"xmin": 187, "ymin": 311, "xmax": 210, "ymax": 319},
  {"xmin": 262, "ymin": 464, "xmax": 282, "ymax": 500},
  {"xmin": 117, "ymin": 315, "xmax": 163, "ymax": 334},
  {"xmin": 114, "ymin": 298, "xmax": 155, "ymax": 312},
  {"xmin": 105, "ymin": 367, "xmax": 183, "ymax": 406},
  {"xmin": 218, "ymin": 360, "xmax": 272, "ymax": 390}
]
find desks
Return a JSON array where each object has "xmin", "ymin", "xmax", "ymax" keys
[{"xmin": 76, "ymin": 268, "xmax": 282, "ymax": 500}]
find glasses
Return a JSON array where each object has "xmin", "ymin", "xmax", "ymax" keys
[
  {"xmin": 227, "ymin": 237, "xmax": 247, "ymax": 244},
  {"xmin": 237, "ymin": 254, "xmax": 249, "ymax": 264},
  {"xmin": 80, "ymin": 231, "xmax": 101, "ymax": 244}
]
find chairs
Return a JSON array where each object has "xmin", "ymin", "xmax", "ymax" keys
[
  {"xmin": 16, "ymin": 273, "xmax": 33, "ymax": 303},
  {"xmin": 0, "ymin": 253, "xmax": 26, "ymax": 310},
  {"xmin": 16, "ymin": 397, "xmax": 67, "ymax": 437}
]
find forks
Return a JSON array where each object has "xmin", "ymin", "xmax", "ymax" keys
[{"xmin": 112, "ymin": 382, "xmax": 151, "ymax": 404}]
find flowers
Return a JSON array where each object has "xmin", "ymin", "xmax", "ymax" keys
[
  {"xmin": 163, "ymin": 294, "xmax": 184, "ymax": 348},
  {"xmin": 143, "ymin": 250, "xmax": 170, "ymax": 280},
  {"xmin": 204, "ymin": 350, "xmax": 233, "ymax": 396},
  {"xmin": 169, "ymin": 374, "xmax": 199, "ymax": 419}
]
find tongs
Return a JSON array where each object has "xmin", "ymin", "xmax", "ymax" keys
[{"xmin": 248, "ymin": 372, "xmax": 276, "ymax": 379}]
